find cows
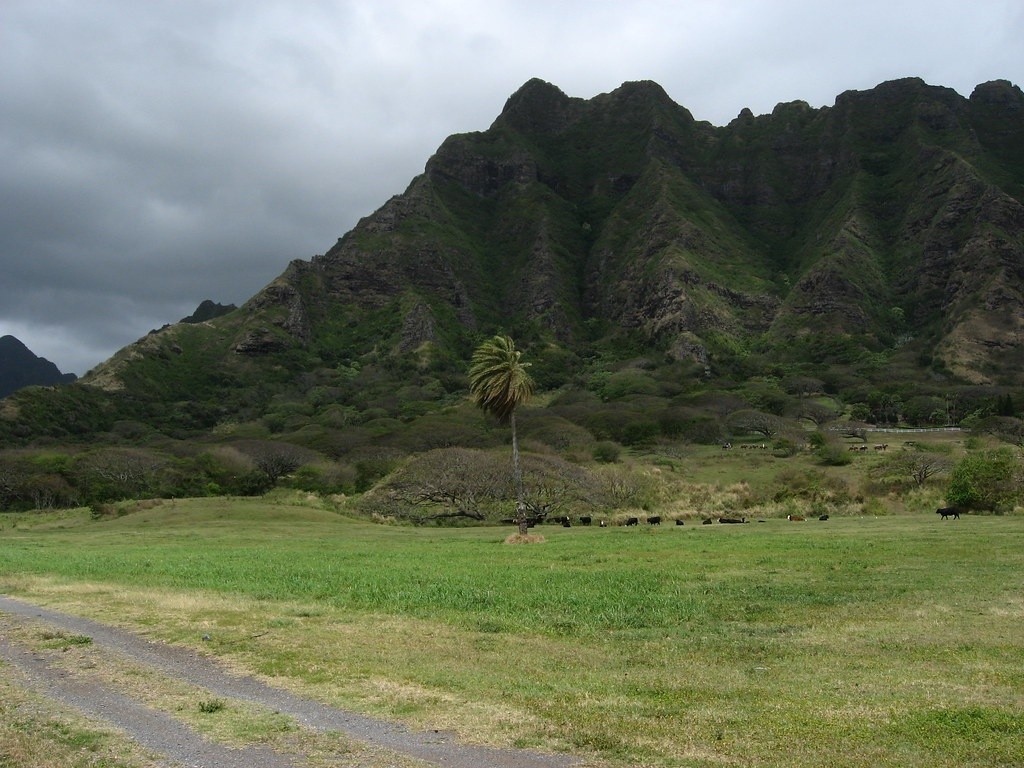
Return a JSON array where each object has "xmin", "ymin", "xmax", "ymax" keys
[
  {"xmin": 847, "ymin": 444, "xmax": 888, "ymax": 452},
  {"xmin": 498, "ymin": 513, "xmax": 830, "ymax": 525},
  {"xmin": 936, "ymin": 507, "xmax": 962, "ymax": 520},
  {"xmin": 721, "ymin": 441, "xmax": 767, "ymax": 451}
]
